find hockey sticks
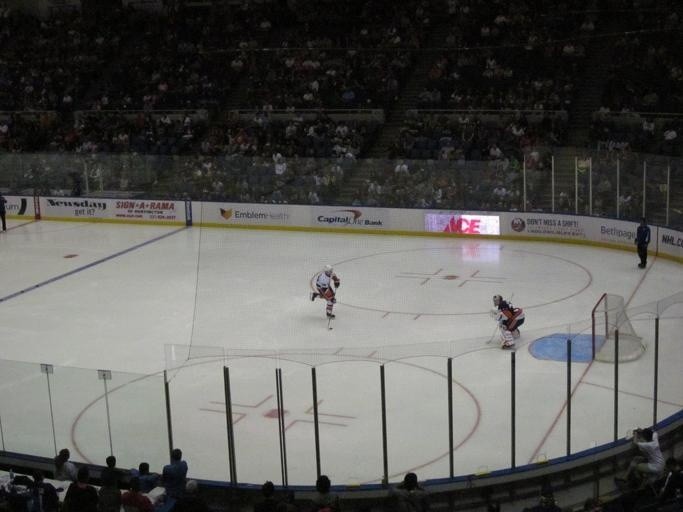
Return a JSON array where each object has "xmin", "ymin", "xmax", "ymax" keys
[
  {"xmin": 327, "ymin": 286, "xmax": 337, "ymax": 330},
  {"xmin": 485, "ymin": 293, "xmax": 515, "ymax": 344}
]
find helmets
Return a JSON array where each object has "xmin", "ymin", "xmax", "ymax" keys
[
  {"xmin": 322, "ymin": 265, "xmax": 331, "ymax": 272},
  {"xmin": 493, "ymin": 296, "xmax": 503, "ymax": 307}
]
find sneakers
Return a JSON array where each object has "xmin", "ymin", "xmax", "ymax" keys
[
  {"xmin": 327, "ymin": 312, "xmax": 334, "ymax": 317},
  {"xmin": 502, "ymin": 344, "xmax": 514, "ymax": 349},
  {"xmin": 312, "ymin": 293, "xmax": 317, "ymax": 301}
]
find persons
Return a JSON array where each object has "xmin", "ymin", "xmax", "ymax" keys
[
  {"xmin": 0, "ymin": 0, "xmax": 683, "ymax": 233},
  {"xmin": 312, "ymin": 265, "xmax": 340, "ymax": 319},
  {"xmin": 0, "ymin": 427, "xmax": 683, "ymax": 512},
  {"xmin": 635, "ymin": 217, "xmax": 650, "ymax": 268},
  {"xmin": 493, "ymin": 295, "xmax": 525, "ymax": 348}
]
